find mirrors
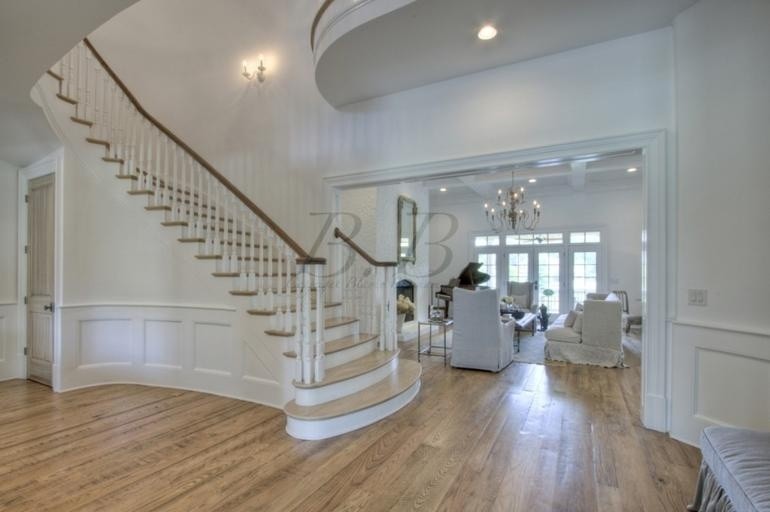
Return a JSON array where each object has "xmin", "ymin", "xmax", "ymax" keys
[{"xmin": 397, "ymin": 194, "xmax": 418, "ymax": 265}]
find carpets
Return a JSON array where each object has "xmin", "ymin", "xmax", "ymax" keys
[
  {"xmin": 621, "ymin": 328, "xmax": 642, "ymax": 357},
  {"xmin": 405, "ymin": 320, "xmax": 567, "ymax": 369}
]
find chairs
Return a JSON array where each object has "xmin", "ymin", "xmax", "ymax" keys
[
  {"xmin": 506, "ymin": 278, "xmax": 539, "ymax": 333},
  {"xmin": 612, "ymin": 290, "xmax": 630, "ymax": 334},
  {"xmin": 448, "ymin": 284, "xmax": 519, "ymax": 375}
]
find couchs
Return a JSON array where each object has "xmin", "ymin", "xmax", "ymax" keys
[{"xmin": 542, "ymin": 289, "xmax": 629, "ymax": 370}]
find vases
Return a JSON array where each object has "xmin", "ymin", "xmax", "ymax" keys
[{"xmin": 396, "ymin": 313, "xmax": 407, "ymax": 341}]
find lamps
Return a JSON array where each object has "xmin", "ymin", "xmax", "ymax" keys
[
  {"xmin": 240, "ymin": 55, "xmax": 268, "ymax": 87},
  {"xmin": 482, "ymin": 167, "xmax": 543, "ymax": 238}
]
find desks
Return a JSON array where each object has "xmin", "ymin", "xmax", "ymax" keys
[
  {"xmin": 503, "ymin": 311, "xmax": 538, "ymax": 353},
  {"xmin": 416, "ymin": 318, "xmax": 454, "ymax": 370}
]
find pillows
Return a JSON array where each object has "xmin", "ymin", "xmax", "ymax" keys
[
  {"xmin": 514, "ymin": 294, "xmax": 529, "ymax": 309},
  {"xmin": 562, "ymin": 308, "xmax": 577, "ymax": 328},
  {"xmin": 575, "ymin": 302, "xmax": 584, "ymax": 312},
  {"xmin": 574, "ymin": 309, "xmax": 584, "ymax": 333}
]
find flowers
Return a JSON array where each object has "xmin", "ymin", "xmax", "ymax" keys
[
  {"xmin": 397, "ymin": 292, "xmax": 419, "ymax": 315},
  {"xmin": 502, "ymin": 296, "xmax": 514, "ymax": 306}
]
